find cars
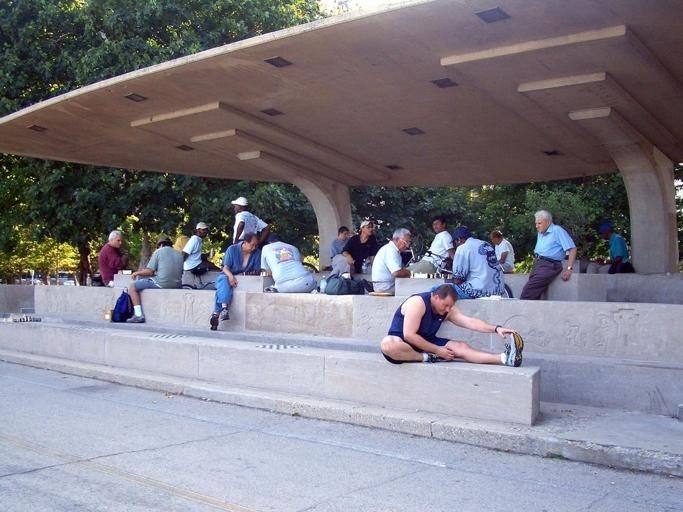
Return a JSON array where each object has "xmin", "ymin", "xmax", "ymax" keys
[{"xmin": 18, "ymin": 271, "xmax": 100, "ymax": 285}]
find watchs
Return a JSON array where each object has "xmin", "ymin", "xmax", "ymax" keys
[
  {"xmin": 494, "ymin": 325, "xmax": 502, "ymax": 333},
  {"xmin": 565, "ymin": 265, "xmax": 573, "ymax": 270}
]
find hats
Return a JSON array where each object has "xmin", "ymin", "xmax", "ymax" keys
[
  {"xmin": 597, "ymin": 224, "xmax": 611, "ymax": 233},
  {"xmin": 450, "ymin": 227, "xmax": 470, "ymax": 242},
  {"xmin": 360, "ymin": 220, "xmax": 372, "ymax": 226},
  {"xmin": 229, "ymin": 198, "xmax": 248, "ymax": 206},
  {"xmin": 195, "ymin": 221, "xmax": 208, "ymax": 230},
  {"xmin": 156, "ymin": 238, "xmax": 171, "ymax": 244}
]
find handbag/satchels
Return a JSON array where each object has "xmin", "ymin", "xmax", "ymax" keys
[
  {"xmin": 191, "ymin": 268, "xmax": 206, "ymax": 275},
  {"xmin": 608, "ymin": 263, "xmax": 634, "ymax": 273},
  {"xmin": 325, "ymin": 275, "xmax": 364, "ymax": 294},
  {"xmin": 111, "ymin": 287, "xmax": 134, "ymax": 322}
]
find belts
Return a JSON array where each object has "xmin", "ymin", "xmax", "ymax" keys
[{"xmin": 533, "ymin": 254, "xmax": 561, "ymax": 264}]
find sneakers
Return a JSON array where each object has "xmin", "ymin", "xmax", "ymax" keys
[
  {"xmin": 127, "ymin": 315, "xmax": 145, "ymax": 323},
  {"xmin": 221, "ymin": 307, "xmax": 229, "ymax": 322},
  {"xmin": 210, "ymin": 315, "xmax": 219, "ymax": 330},
  {"xmin": 504, "ymin": 332, "xmax": 523, "ymax": 366},
  {"xmin": 425, "ymin": 349, "xmax": 452, "ymax": 362}
]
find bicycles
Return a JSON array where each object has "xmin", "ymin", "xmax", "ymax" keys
[
  {"xmin": 180, "ymin": 268, "xmax": 216, "ymax": 290},
  {"xmin": 422, "ymin": 250, "xmax": 512, "ymax": 298}
]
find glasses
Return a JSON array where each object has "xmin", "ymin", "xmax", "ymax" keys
[{"xmin": 398, "ymin": 238, "xmax": 412, "ymax": 245}]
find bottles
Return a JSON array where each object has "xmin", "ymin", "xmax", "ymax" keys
[
  {"xmin": 86, "ymin": 273, "xmax": 91, "ymax": 286},
  {"xmin": 361, "ymin": 258, "xmax": 367, "ymax": 274},
  {"xmin": 320, "ymin": 276, "xmax": 326, "ymax": 293}
]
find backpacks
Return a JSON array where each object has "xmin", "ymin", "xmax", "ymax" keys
[{"xmin": 113, "ymin": 290, "xmax": 134, "ymax": 321}]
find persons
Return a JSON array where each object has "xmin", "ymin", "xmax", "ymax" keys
[
  {"xmin": 373, "ymin": 229, "xmax": 411, "ymax": 292},
  {"xmin": 430, "ymin": 228, "xmax": 505, "ymax": 300},
  {"xmin": 586, "ymin": 221, "xmax": 629, "ymax": 275},
  {"xmin": 209, "ymin": 232, "xmax": 262, "ymax": 331},
  {"xmin": 490, "ymin": 231, "xmax": 515, "ymax": 272},
  {"xmin": 127, "ymin": 236, "xmax": 184, "ymax": 323},
  {"xmin": 330, "ymin": 226, "xmax": 351, "ymax": 270},
  {"xmin": 406, "ymin": 215, "xmax": 454, "ymax": 277},
  {"xmin": 262, "ymin": 234, "xmax": 324, "ymax": 293},
  {"xmin": 381, "ymin": 285, "xmax": 523, "ymax": 367},
  {"xmin": 326, "ymin": 221, "xmax": 380, "ymax": 284},
  {"xmin": 182, "ymin": 222, "xmax": 220, "ymax": 276},
  {"xmin": 231, "ymin": 198, "xmax": 270, "ymax": 244},
  {"xmin": 99, "ymin": 231, "xmax": 137, "ymax": 286},
  {"xmin": 520, "ymin": 210, "xmax": 577, "ymax": 300}
]
